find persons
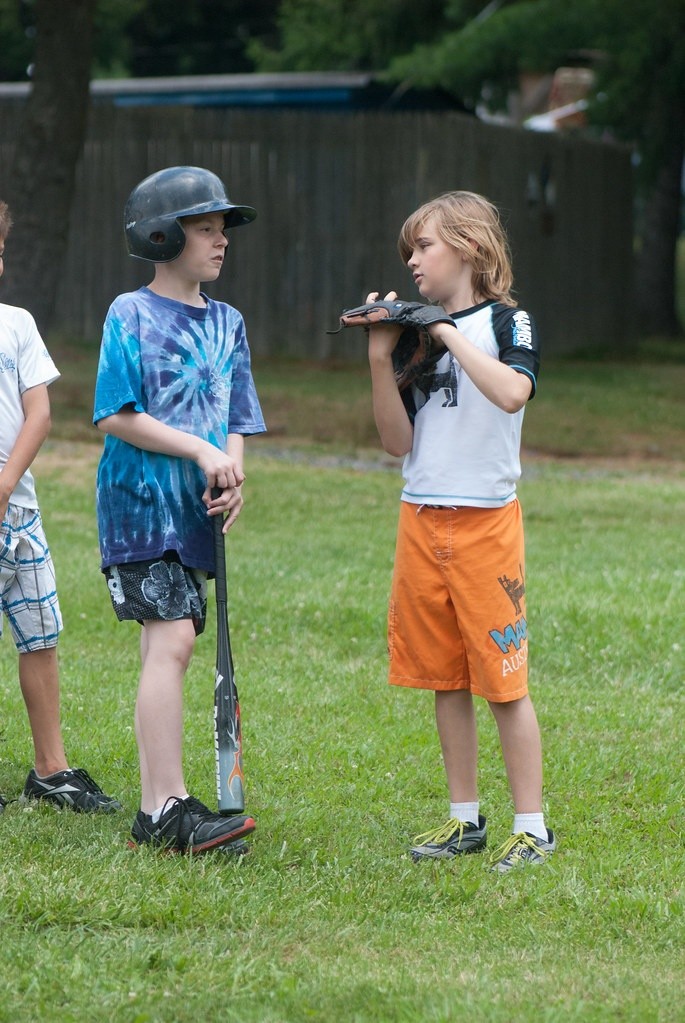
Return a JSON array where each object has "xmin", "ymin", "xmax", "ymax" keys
[
  {"xmin": 92, "ymin": 166, "xmax": 268, "ymax": 857},
  {"xmin": 0, "ymin": 200, "xmax": 124, "ymax": 815},
  {"xmin": 325, "ymin": 190, "xmax": 557, "ymax": 873}
]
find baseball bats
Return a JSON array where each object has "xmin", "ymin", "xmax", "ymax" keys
[{"xmin": 212, "ymin": 471, "xmax": 245, "ymax": 812}]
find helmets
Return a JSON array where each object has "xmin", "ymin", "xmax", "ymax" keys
[{"xmin": 123, "ymin": 166, "xmax": 258, "ymax": 263}]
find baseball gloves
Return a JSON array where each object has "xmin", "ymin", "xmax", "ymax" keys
[{"xmin": 339, "ymin": 297, "xmax": 457, "ymax": 391}]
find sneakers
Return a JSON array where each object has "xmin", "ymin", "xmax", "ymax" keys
[
  {"xmin": 204, "ymin": 839, "xmax": 252, "ymax": 860},
  {"xmin": 128, "ymin": 795, "xmax": 256, "ymax": 856},
  {"xmin": 409, "ymin": 814, "xmax": 487, "ymax": 864},
  {"xmin": 0, "ymin": 793, "xmax": 18, "ymax": 817},
  {"xmin": 18, "ymin": 767, "xmax": 121, "ymax": 816},
  {"xmin": 490, "ymin": 827, "xmax": 556, "ymax": 877}
]
